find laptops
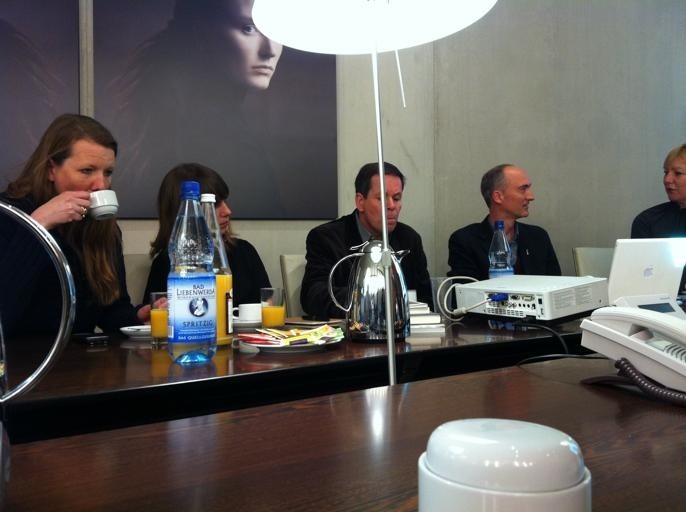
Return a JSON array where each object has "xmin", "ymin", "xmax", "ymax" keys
[{"xmin": 607, "ymin": 238, "xmax": 686, "ymax": 305}]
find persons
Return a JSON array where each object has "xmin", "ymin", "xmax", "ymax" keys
[
  {"xmin": 143, "ymin": 165, "xmax": 273, "ymax": 315},
  {"xmin": 631, "ymin": 144, "xmax": 686, "ymax": 239},
  {"xmin": 447, "ymin": 164, "xmax": 563, "ymax": 310},
  {"xmin": 300, "ymin": 162, "xmax": 434, "ymax": 324},
  {"xmin": 0, "ymin": 116, "xmax": 166, "ymax": 343},
  {"xmin": 108, "ymin": 0, "xmax": 282, "ymax": 218}
]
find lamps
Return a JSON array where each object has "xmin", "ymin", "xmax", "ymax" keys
[{"xmin": 249, "ymin": 0, "xmax": 499, "ymax": 384}]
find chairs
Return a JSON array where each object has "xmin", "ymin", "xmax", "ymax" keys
[
  {"xmin": 122, "ymin": 253, "xmax": 156, "ymax": 308},
  {"xmin": 279, "ymin": 253, "xmax": 307, "ymax": 318},
  {"xmin": 571, "ymin": 245, "xmax": 615, "ymax": 281}
]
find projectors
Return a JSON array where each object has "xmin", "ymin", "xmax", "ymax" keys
[{"xmin": 454, "ymin": 274, "xmax": 609, "ymax": 326}]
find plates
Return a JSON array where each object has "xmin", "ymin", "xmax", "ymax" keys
[
  {"xmin": 233, "ymin": 320, "xmax": 262, "ymax": 330},
  {"xmin": 120, "ymin": 324, "xmax": 151, "ymax": 338},
  {"xmin": 239, "ymin": 334, "xmax": 341, "ymax": 353}
]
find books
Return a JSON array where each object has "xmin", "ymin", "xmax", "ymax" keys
[
  {"xmin": 408, "ymin": 302, "xmax": 446, "ymax": 334},
  {"xmin": 285, "ymin": 316, "xmax": 342, "ymax": 325}
]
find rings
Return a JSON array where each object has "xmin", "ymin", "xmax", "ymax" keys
[{"xmin": 81, "ymin": 207, "xmax": 87, "ymax": 218}]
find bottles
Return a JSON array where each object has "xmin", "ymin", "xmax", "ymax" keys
[
  {"xmin": 489, "ymin": 221, "xmax": 515, "ymax": 278},
  {"xmin": 199, "ymin": 193, "xmax": 234, "ymax": 346},
  {"xmin": 166, "ymin": 181, "xmax": 217, "ymax": 363}
]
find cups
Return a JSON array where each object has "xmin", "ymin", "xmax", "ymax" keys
[
  {"xmin": 429, "ymin": 277, "xmax": 453, "ymax": 320},
  {"xmin": 260, "ymin": 288, "xmax": 286, "ymax": 329},
  {"xmin": 233, "ymin": 304, "xmax": 261, "ymax": 320},
  {"xmin": 89, "ymin": 190, "xmax": 119, "ymax": 221},
  {"xmin": 150, "ymin": 292, "xmax": 168, "ymax": 344}
]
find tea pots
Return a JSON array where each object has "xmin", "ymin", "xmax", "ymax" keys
[{"xmin": 328, "ymin": 236, "xmax": 410, "ymax": 344}]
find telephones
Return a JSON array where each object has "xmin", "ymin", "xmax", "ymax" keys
[{"xmin": 580, "ymin": 293, "xmax": 686, "ymax": 405}]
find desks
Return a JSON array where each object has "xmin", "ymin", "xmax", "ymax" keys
[
  {"xmin": 0, "ymin": 315, "xmax": 584, "ymax": 438},
  {"xmin": 0, "ymin": 351, "xmax": 686, "ymax": 512}
]
